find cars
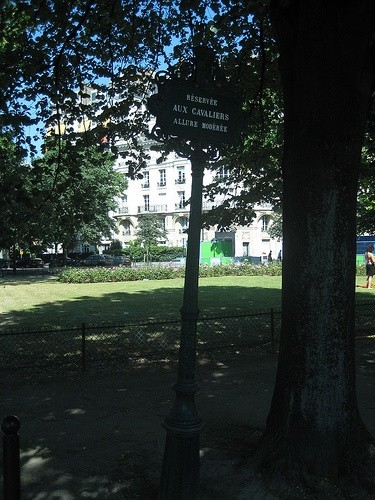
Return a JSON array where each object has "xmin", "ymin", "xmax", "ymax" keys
[
  {"xmin": 83, "ymin": 255, "xmax": 131, "ymax": 267},
  {"xmin": 12, "ymin": 257, "xmax": 44, "ymax": 268},
  {"xmin": 49, "ymin": 256, "xmax": 81, "ymax": 269}
]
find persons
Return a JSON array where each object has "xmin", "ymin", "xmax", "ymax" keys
[
  {"xmin": 364, "ymin": 245, "xmax": 375, "ymax": 288},
  {"xmin": 277, "ymin": 250, "xmax": 282, "ymax": 261},
  {"xmin": 267, "ymin": 250, "xmax": 272, "ymax": 262}
]
